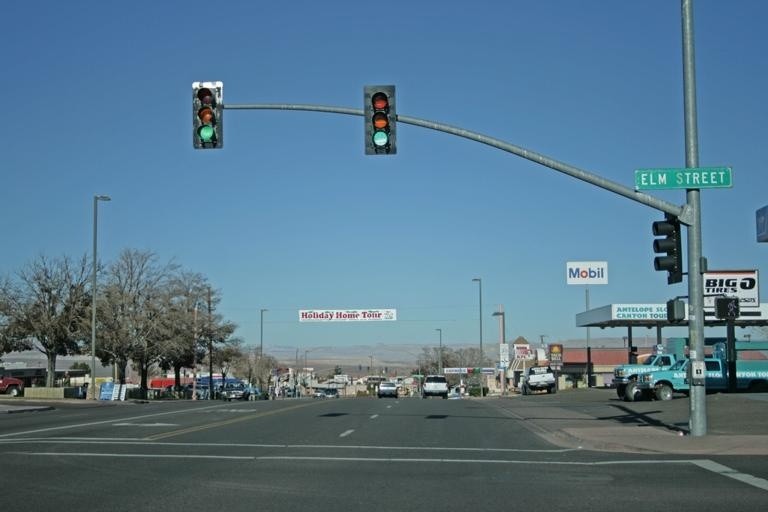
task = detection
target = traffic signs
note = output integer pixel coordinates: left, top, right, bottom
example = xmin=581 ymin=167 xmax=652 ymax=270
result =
xmin=632 ymin=165 xmax=734 ymax=192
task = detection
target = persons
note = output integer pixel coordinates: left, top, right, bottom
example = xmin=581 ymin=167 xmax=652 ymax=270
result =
xmin=64 ymin=372 xmax=70 ymax=386
xmin=31 ymin=377 xmax=40 ymax=387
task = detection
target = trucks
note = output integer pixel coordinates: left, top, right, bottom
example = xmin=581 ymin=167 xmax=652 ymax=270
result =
xmin=633 ymin=340 xmax=767 ymax=402
xmin=609 ymin=336 xmax=737 ymax=402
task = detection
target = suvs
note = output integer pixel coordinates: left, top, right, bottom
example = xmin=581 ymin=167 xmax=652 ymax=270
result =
xmin=519 ymin=362 xmax=557 ymax=396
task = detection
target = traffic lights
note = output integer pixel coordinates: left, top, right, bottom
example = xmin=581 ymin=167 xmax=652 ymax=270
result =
xmin=664 ymin=298 xmax=685 ymax=324
xmin=713 ymin=296 xmax=740 ymax=320
xmin=650 ymin=210 xmax=682 ymax=285
xmin=190 ymin=80 xmax=225 ymax=151
xmin=362 ymin=83 xmax=397 ymax=155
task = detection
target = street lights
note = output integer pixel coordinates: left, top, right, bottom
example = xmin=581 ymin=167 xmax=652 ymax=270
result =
xmin=204 ymin=284 xmax=301 ymax=401
xmin=86 ymin=193 xmax=112 ymax=403
xmin=365 ymin=274 xmax=483 ymax=401
